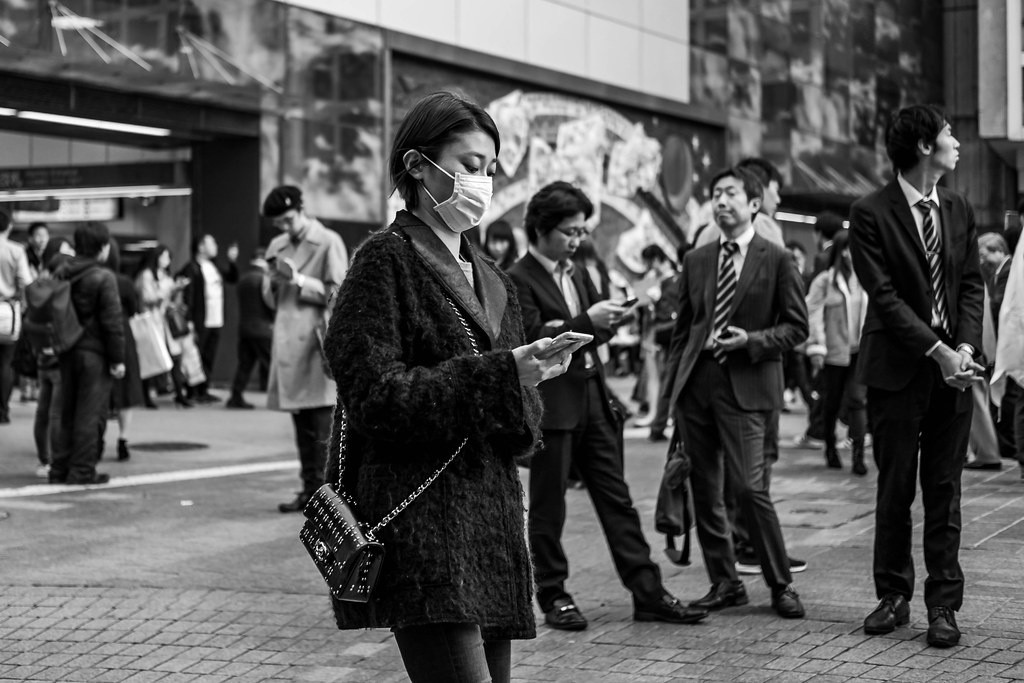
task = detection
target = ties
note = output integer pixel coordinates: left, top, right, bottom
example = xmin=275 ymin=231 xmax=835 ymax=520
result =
xmin=711 ymin=242 xmax=740 ymax=364
xmin=915 ymin=199 xmax=952 ymax=339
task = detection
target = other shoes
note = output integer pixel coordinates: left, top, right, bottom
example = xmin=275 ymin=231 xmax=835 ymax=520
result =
xmin=963 ymin=460 xmax=1003 ymax=471
xmin=141 ymin=387 xmax=254 ymax=410
xmin=36 ymin=464 xmax=50 ymax=477
xmin=49 ymin=469 xmax=110 ymax=486
xmin=118 ymin=439 xmax=131 ymax=460
xmin=825 ymin=448 xmax=842 ymax=469
xmin=733 ymin=554 xmax=808 ymax=573
xmin=850 ymin=449 xmax=867 ymax=476
xmin=279 ymin=496 xmax=307 ymax=512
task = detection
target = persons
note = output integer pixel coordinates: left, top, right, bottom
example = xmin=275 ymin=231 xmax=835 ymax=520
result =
xmin=849 ymin=103 xmax=984 ymax=645
xmin=484 ymin=181 xmax=707 ymax=630
xmin=967 ymin=217 xmax=1024 ymax=478
xmin=0 ymin=206 xmax=274 ymax=484
xmin=324 ymin=89 xmax=571 ymax=682
xmin=252 ymin=185 xmax=350 ymax=512
xmin=651 ymin=155 xmax=871 ymax=616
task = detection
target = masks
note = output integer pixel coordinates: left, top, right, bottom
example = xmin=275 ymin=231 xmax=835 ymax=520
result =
xmin=413 ymin=153 xmax=494 ymax=232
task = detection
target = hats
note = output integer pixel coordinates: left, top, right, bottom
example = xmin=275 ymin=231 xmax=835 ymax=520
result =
xmin=263 ymin=186 xmax=301 ymax=216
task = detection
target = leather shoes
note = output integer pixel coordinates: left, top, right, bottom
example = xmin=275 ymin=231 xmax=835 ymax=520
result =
xmin=545 ymin=597 xmax=587 ymax=630
xmin=772 ymin=584 xmax=805 ymax=619
xmin=863 ymin=592 xmax=909 ymax=634
xmin=632 ymin=588 xmax=709 ymax=623
xmin=928 ymin=604 xmax=960 ymax=647
xmin=689 ymin=580 xmax=750 ymax=612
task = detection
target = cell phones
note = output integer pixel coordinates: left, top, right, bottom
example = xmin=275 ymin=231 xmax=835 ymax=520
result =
xmin=266 ymin=255 xmax=277 ymax=269
xmin=533 ymin=331 xmax=595 ymax=365
xmin=718 ymin=329 xmax=732 ymax=340
xmin=615 ymin=297 xmax=639 ymax=317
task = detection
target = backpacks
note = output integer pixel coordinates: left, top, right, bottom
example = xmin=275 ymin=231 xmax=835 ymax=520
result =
xmin=26 ymin=259 xmax=98 ymax=357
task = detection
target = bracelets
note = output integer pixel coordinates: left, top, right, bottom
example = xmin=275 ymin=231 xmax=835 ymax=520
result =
xmin=960 ymin=345 xmax=973 ymax=355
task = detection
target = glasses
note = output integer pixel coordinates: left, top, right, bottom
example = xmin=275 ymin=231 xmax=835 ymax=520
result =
xmin=553 ymin=225 xmax=590 ymax=243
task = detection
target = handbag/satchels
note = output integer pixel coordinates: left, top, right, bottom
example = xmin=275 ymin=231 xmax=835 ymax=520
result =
xmin=299 ymin=487 xmax=385 ymax=602
xmin=0 ymin=297 xmax=23 ymax=343
xmin=655 ymin=420 xmax=697 ymax=564
